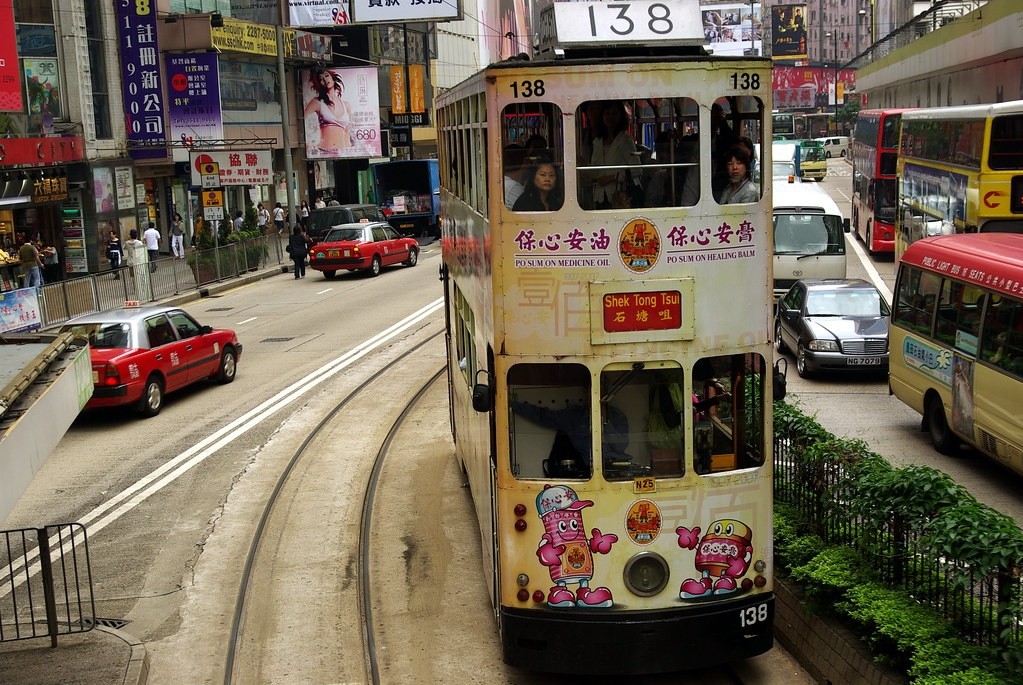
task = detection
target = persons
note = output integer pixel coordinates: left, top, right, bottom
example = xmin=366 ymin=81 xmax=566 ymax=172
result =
xmin=304 ymin=66 xmax=356 ymax=157
xmin=300 ymin=200 xmax=311 ymax=236
xmin=143 ymin=221 xmax=161 ymax=273
xmin=314 ymin=196 xmax=326 ymax=209
xmin=288 ymin=225 xmax=312 ymax=279
xmin=255 ymin=203 xmax=271 ymax=236
xmin=272 ymin=202 xmax=300 ymax=237
xmin=234 ymin=210 xmax=244 ymax=230
xmin=794 ymin=9 xmax=803 ymax=25
xmin=194 ymin=215 xmax=204 ymax=235
xmin=959 ymin=290 xmax=1022 ymax=370
xmin=644 ymin=376 xmax=734 ymax=469
xmin=122 ymin=229 xmax=143 ymax=292
xmin=327 ymin=194 xmax=340 ymax=206
xmin=0 ymin=233 xmax=60 ymax=289
xmin=901 ymin=292 xmax=957 ymax=333
xmin=105 ymin=230 xmax=124 ymax=281
xmin=366 ymin=182 xmax=375 ymax=203
xmin=314 ymin=162 xmax=322 ymax=189
xmin=224 ymin=212 xmax=234 ymax=234
xmin=514 ymin=369 xmax=629 ymax=472
xmin=168 ymin=213 xmax=187 ymax=261
xmin=774 ymin=9 xmax=788 ymax=31
xmin=504 ymin=102 xmax=764 ymax=212
xmin=105 ymin=182 xmax=114 ymax=210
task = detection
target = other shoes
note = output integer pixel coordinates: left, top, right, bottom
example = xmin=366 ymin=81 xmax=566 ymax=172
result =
xmin=151 ymin=268 xmax=157 ymax=273
xmin=114 ymin=275 xmax=120 ymax=279
xmin=277 ymin=232 xmax=281 ymax=238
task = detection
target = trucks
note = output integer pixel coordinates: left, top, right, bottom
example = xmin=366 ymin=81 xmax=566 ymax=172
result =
xmin=372 ymin=158 xmax=441 ymax=235
xmin=794 ymin=139 xmax=827 ymax=182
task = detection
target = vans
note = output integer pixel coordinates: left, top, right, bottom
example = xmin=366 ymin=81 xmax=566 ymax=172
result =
xmin=752 ymin=141 xmax=797 ymax=183
xmin=306 ymin=203 xmax=391 ymax=242
xmin=815 ymin=136 xmax=848 ymax=159
xmin=889 ymin=232 xmax=1023 ymax=480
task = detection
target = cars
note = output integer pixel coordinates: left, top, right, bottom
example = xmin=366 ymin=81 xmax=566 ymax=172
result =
xmin=309 ymin=219 xmax=420 ymax=280
xmin=55 ymin=301 xmax=243 ymax=417
xmin=776 ymin=277 xmax=892 ymax=379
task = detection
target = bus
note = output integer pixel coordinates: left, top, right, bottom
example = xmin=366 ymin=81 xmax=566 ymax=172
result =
xmin=433 ymin=37 xmax=789 ymax=678
xmin=754 ymin=160 xmax=851 ymax=314
xmin=893 ymin=101 xmax=1023 ymax=287
xmin=850 ymin=107 xmax=924 ymax=256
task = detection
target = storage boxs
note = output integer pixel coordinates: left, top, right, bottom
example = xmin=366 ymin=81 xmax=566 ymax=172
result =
xmin=391 ymin=195 xmax=408 ymax=212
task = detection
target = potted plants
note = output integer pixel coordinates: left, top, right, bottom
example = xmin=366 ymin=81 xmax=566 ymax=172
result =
xmin=185 ymin=201 xmax=269 ymax=285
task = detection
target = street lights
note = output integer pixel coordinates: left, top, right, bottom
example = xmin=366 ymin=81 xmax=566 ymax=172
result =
xmin=858 ymin=4 xmax=874 ymax=63
xmin=825 ymin=28 xmax=838 ymax=136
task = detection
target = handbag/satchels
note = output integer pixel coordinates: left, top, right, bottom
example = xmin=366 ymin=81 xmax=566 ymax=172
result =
xmin=265 ymin=221 xmax=269 ymax=229
xmin=611 ymin=168 xmax=647 ymax=209
xmin=274 ymin=220 xmax=276 ymax=225
